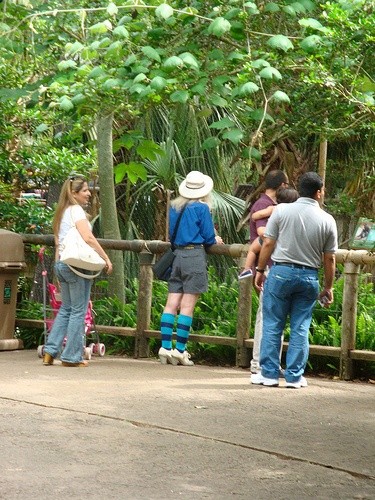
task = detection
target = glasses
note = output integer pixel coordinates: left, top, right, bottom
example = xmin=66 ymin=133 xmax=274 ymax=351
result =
xmin=279 ymin=183 xmax=290 ymax=186
xmin=70 ymin=178 xmax=74 ymax=192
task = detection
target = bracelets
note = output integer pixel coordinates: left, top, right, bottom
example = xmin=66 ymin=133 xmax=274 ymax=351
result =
xmin=255 ymin=267 xmax=264 ymax=272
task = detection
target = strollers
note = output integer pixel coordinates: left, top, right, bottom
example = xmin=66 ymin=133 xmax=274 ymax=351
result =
xmin=38 ymin=248 xmax=105 ymax=359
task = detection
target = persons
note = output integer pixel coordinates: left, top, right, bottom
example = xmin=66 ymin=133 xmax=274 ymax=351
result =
xmin=254 ymin=172 xmax=338 ymax=388
xmin=249 ymin=169 xmax=289 ymax=377
xmin=238 ymin=186 xmax=300 ymax=279
xmin=43 ymin=178 xmax=113 ymax=367
xmin=158 ymin=172 xmax=222 ymax=366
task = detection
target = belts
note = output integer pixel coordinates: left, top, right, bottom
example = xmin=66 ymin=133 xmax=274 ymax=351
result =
xmin=272 ymin=261 xmax=311 ymax=269
xmin=174 ymin=244 xmax=204 ymax=250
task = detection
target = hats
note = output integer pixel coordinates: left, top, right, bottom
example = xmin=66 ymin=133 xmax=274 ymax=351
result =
xmin=179 ymin=171 xmax=214 ymax=199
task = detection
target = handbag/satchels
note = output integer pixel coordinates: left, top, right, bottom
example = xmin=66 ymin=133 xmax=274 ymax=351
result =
xmin=60 ymin=205 xmax=106 ymax=271
xmin=153 ymin=250 xmax=176 ymax=281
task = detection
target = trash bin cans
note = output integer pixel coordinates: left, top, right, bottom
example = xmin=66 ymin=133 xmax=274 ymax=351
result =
xmin=0 ymin=228 xmax=25 ymax=350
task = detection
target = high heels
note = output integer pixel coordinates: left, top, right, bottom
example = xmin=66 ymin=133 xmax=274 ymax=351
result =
xmin=171 ymin=348 xmax=194 ymax=366
xmin=158 ymin=347 xmax=181 ymax=365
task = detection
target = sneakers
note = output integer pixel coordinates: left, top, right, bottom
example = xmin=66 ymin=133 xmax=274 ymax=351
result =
xmin=251 ymin=374 xmax=279 ymax=386
xmin=287 ymin=375 xmax=307 ymax=388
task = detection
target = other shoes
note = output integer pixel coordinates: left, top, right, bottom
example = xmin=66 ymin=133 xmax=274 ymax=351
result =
xmin=43 ymin=353 xmax=54 ymax=365
xmin=62 ymin=361 xmax=86 ymax=367
xmin=238 ymin=269 xmax=253 ymax=279
